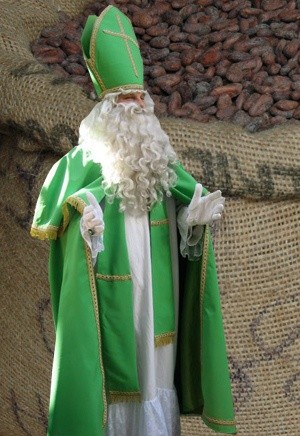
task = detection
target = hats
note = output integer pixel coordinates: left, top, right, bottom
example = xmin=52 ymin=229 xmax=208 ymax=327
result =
xmin=80 ymin=5 xmax=144 ymax=99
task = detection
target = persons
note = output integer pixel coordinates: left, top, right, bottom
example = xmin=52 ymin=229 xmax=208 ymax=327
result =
xmin=30 ymin=5 xmax=237 ymax=435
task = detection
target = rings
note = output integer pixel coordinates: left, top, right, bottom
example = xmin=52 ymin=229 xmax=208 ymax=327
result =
xmin=88 ymin=227 xmax=95 ymax=235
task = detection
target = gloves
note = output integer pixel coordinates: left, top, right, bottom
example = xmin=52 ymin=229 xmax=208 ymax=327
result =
xmin=84 ymin=191 xmax=104 ymax=237
xmin=188 ymin=183 xmax=225 ymax=225
